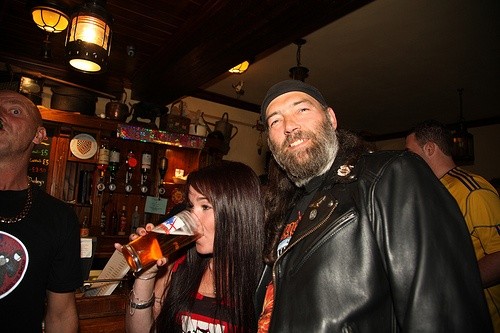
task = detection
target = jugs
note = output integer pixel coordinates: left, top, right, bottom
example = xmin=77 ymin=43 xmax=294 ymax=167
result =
xmin=202 ymin=111 xmax=239 ymax=144
xmin=105 ymin=89 xmax=135 ymax=124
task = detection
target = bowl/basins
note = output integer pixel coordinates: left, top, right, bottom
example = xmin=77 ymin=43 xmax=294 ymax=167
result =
xmin=172 ymin=176 xmax=188 ymax=184
xmin=188 ymin=123 xmax=207 ymax=137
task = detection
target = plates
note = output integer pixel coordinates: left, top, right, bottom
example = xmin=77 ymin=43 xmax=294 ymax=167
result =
xmin=69 ymin=133 xmax=98 ymax=159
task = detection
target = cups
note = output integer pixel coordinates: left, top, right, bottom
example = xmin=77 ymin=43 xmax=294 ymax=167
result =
xmin=121 ymin=207 xmax=204 ymax=272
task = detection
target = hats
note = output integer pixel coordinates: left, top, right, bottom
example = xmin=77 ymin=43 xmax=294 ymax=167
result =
xmin=261 ymin=80 xmax=327 ymax=114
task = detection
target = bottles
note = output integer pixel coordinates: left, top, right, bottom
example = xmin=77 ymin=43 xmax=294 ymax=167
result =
xmin=123 ymin=151 xmax=138 ymax=197
xmin=141 ymin=152 xmax=151 ymax=197
xmin=110 ymin=202 xmax=118 ymax=236
xmin=100 ymin=208 xmax=106 ymax=235
xmin=80 ymin=215 xmax=89 ymax=238
xmin=97 ymin=144 xmax=110 ymax=195
xmin=108 ymin=148 xmax=120 ymax=196
xmin=118 ymin=206 xmax=127 ymax=235
xmin=131 ymin=206 xmax=148 ymax=234
xmin=158 ymin=157 xmax=168 ymax=198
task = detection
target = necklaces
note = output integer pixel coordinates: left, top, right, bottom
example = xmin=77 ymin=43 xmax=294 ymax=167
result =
xmin=207 ymin=261 xmax=218 ymax=296
xmin=0 ymin=175 xmax=33 ymax=223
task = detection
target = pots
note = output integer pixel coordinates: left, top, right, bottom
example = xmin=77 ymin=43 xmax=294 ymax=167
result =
xmin=50 ymin=86 xmax=97 ymax=114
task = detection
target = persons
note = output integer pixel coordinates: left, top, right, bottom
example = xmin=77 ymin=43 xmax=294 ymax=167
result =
xmin=400 ymin=112 xmax=500 ymax=333
xmin=115 ymin=160 xmax=275 ymax=333
xmin=261 ymin=78 xmax=479 ymax=333
xmin=0 ymin=89 xmax=79 ymax=333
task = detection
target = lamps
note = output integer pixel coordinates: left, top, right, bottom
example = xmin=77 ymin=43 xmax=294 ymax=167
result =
xmin=448 ymin=89 xmax=475 ymax=166
xmin=30 ymin=0 xmax=70 ymax=34
xmin=64 ymin=0 xmax=112 ymax=74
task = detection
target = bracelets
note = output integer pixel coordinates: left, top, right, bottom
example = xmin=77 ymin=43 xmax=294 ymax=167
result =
xmin=128 ymin=289 xmax=156 ymax=315
xmin=133 ymin=272 xmax=158 ymax=281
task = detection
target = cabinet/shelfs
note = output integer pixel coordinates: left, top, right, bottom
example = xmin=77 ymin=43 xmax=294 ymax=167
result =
xmin=37 ymin=106 xmax=205 ymax=257
